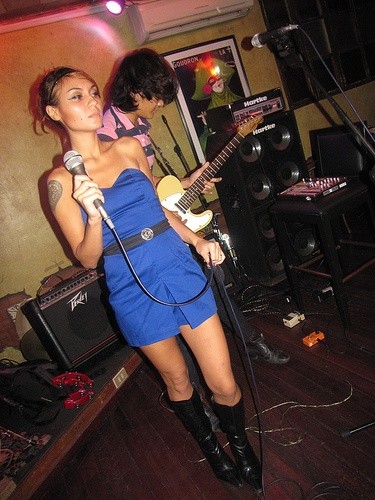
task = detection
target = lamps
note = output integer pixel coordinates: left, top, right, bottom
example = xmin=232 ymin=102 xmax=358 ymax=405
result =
xmin=106 ymin=0 xmax=125 ymax=15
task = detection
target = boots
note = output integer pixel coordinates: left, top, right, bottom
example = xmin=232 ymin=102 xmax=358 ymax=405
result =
xmin=162 ymin=384 xmax=245 ymax=489
xmin=212 ymin=393 xmax=263 ymax=491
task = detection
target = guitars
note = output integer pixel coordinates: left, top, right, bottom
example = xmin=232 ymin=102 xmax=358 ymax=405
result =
xmin=157 ymin=116 xmax=266 ymax=244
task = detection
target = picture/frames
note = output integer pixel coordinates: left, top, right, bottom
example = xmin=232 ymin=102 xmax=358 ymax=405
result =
xmin=160 ymin=34 xmax=253 ymax=166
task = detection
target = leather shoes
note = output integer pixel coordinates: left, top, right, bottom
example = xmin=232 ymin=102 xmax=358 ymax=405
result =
xmin=245 ymin=333 xmax=292 ymax=366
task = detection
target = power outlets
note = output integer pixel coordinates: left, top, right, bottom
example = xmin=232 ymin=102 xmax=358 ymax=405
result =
xmin=112 ymin=367 xmax=129 ymax=389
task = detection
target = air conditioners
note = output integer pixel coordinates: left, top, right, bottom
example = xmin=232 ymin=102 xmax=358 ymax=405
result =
xmin=126 ymin=0 xmax=254 ymax=46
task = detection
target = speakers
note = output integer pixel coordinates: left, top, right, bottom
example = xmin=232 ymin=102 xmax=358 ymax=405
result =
xmin=206 ymin=109 xmax=341 ymax=287
xmin=21 ymin=266 xmax=124 ymax=372
xmin=189 ymin=233 xmax=243 ymax=295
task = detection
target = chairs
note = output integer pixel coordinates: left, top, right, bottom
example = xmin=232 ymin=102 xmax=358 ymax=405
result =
xmin=268 ymin=120 xmax=375 ymax=329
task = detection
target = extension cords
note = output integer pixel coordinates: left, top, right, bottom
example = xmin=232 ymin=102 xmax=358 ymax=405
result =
xmin=318 ymin=287 xmax=334 ymax=303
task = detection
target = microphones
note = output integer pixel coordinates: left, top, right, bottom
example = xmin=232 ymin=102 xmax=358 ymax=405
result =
xmin=64 ymin=151 xmax=115 ymax=229
xmin=242 ymin=23 xmax=299 ymax=50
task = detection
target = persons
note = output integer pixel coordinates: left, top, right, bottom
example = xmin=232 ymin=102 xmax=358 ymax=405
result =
xmin=97 ymin=48 xmax=292 ymax=366
xmin=39 ymin=66 xmax=262 ymax=492
